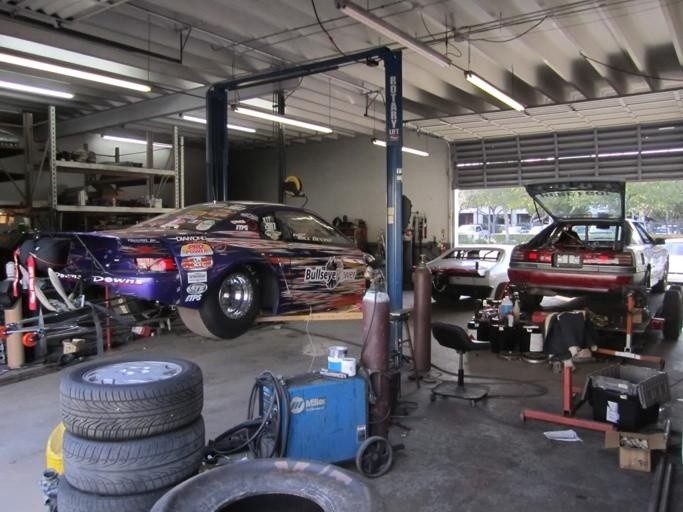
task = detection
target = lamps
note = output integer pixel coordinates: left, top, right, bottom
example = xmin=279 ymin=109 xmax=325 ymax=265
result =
xmin=336 ymin=0 xmax=451 ymax=69
xmin=0 ymin=53 xmax=151 ymax=93
xmin=464 ymin=70 xmax=527 ymax=111
xmin=371 ymin=138 xmax=429 ymax=157
xmin=179 ymin=114 xmax=256 ymax=133
xmin=231 ymin=104 xmax=332 ymax=134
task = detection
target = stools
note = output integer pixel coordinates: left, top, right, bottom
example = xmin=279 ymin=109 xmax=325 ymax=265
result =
xmin=431 ymin=323 xmax=491 ymax=408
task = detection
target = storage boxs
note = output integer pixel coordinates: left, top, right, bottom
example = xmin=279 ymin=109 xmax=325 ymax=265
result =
xmin=605 ymin=431 xmax=666 ymax=472
xmin=582 ymin=364 xmax=671 ymax=432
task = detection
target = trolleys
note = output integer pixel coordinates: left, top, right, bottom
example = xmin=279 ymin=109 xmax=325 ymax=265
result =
xmin=257 ymin=268 xmax=405 ymax=478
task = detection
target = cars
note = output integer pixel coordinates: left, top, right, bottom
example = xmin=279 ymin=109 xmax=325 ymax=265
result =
xmin=456 ymin=222 xmax=547 ymax=240
xmin=509 ymin=181 xmax=670 ymax=307
xmin=664 ymin=237 xmax=682 ymax=285
xmin=588 ymin=225 xmax=612 ymax=240
xmin=425 ymin=243 xmax=515 ymax=302
xmin=13 ymin=200 xmax=376 ymax=339
xmin=652 ymin=223 xmax=680 ymax=235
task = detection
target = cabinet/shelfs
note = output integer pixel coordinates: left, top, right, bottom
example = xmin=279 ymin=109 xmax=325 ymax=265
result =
xmin=48 ymin=106 xmax=184 ymax=214
xmin=0 ymin=113 xmax=33 ymax=208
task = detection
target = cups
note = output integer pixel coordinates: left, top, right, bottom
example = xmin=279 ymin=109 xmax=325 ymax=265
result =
xmin=340 ymin=357 xmax=356 ymax=378
xmin=76 ymin=190 xmax=86 ymax=206
xmin=154 ymin=199 xmax=162 ymax=209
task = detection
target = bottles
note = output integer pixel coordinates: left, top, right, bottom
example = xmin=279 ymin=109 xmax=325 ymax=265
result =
xmin=499 ymin=295 xmax=520 ymax=327
xmin=327 ymin=347 xmax=348 ymax=373
